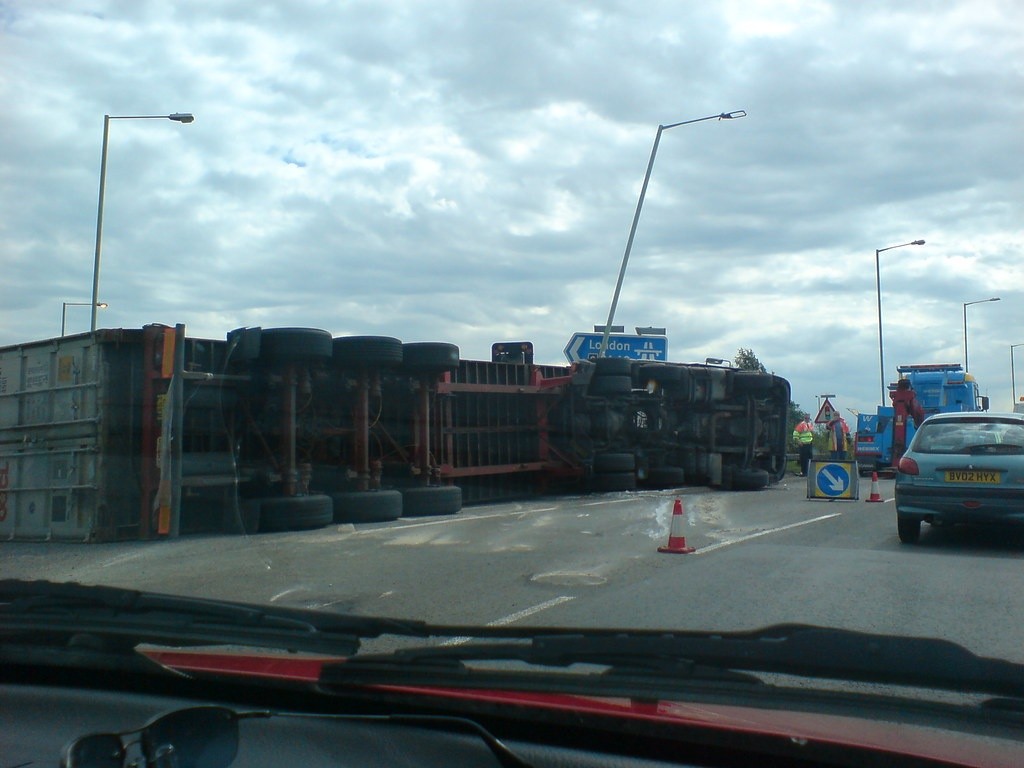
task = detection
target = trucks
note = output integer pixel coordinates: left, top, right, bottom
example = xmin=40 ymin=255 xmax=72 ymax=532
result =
xmin=852 ymin=363 xmax=989 ymax=478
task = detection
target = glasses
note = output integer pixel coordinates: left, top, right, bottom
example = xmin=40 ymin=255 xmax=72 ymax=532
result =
xmin=59 ymin=705 xmax=534 ymax=768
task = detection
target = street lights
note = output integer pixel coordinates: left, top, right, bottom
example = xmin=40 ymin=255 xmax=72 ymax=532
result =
xmin=814 ymin=395 xmax=821 ymax=435
xmin=62 ymin=302 xmax=108 ymax=335
xmin=595 ymin=108 xmax=751 ymax=358
xmin=875 ymin=240 xmax=926 ymax=405
xmin=963 ymin=297 xmax=1001 ymax=374
xmin=90 ymin=111 xmax=195 ymax=328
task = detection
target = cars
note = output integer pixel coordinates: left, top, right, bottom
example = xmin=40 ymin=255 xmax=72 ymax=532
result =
xmin=893 ymin=410 xmax=1024 ymax=544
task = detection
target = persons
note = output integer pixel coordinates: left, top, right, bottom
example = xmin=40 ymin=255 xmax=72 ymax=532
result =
xmin=792 ymin=413 xmax=814 ymax=476
xmin=826 ymin=412 xmax=852 ymax=461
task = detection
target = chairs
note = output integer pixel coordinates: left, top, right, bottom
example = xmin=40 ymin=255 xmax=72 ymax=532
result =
xmin=941 ymin=426 xmax=964 ymax=446
xmin=1002 ymin=429 xmax=1024 ymax=446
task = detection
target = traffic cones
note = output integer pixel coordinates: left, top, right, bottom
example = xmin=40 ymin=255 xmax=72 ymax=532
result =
xmin=865 ymin=472 xmax=885 ymax=504
xmin=658 ymin=497 xmax=696 ymax=553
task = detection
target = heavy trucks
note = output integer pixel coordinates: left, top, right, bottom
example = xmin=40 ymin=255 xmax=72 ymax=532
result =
xmin=0 ymin=324 xmax=793 ymax=542
